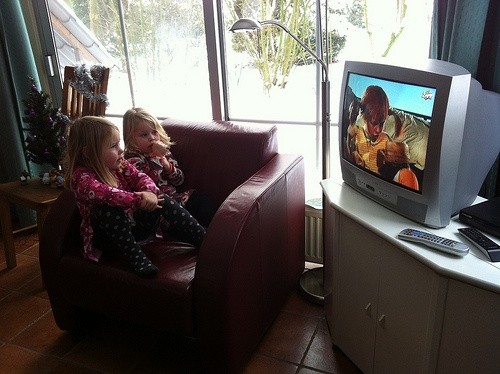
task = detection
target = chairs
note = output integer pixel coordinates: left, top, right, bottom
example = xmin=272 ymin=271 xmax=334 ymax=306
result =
xmin=0 ymin=65 xmax=110 ymax=271
xmin=37 ymin=117 xmax=305 ymax=374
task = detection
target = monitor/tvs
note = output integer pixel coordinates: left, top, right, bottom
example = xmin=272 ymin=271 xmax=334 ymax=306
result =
xmin=337 ymin=58 xmax=500 ymax=228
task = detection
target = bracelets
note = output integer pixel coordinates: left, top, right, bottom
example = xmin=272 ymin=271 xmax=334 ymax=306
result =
xmin=167 ymin=163 xmax=173 ymax=173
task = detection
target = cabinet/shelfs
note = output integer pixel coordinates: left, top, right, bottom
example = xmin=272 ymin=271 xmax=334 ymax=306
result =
xmin=320 ymin=178 xmax=500 ymax=374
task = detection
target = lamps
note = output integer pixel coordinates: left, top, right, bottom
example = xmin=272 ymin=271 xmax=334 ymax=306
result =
xmin=228 ymin=18 xmax=331 ymax=308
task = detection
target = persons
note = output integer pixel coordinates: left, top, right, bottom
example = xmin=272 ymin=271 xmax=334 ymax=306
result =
xmin=347 ymin=85 xmax=420 ymax=191
xmin=122 ymin=108 xmax=216 ymax=228
xmin=63 ymin=116 xmax=207 ymax=279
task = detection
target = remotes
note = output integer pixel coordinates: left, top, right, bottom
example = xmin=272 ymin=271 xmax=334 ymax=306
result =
xmin=458 ymin=228 xmax=500 ymax=261
xmin=398 ymin=229 xmax=469 ymax=256
